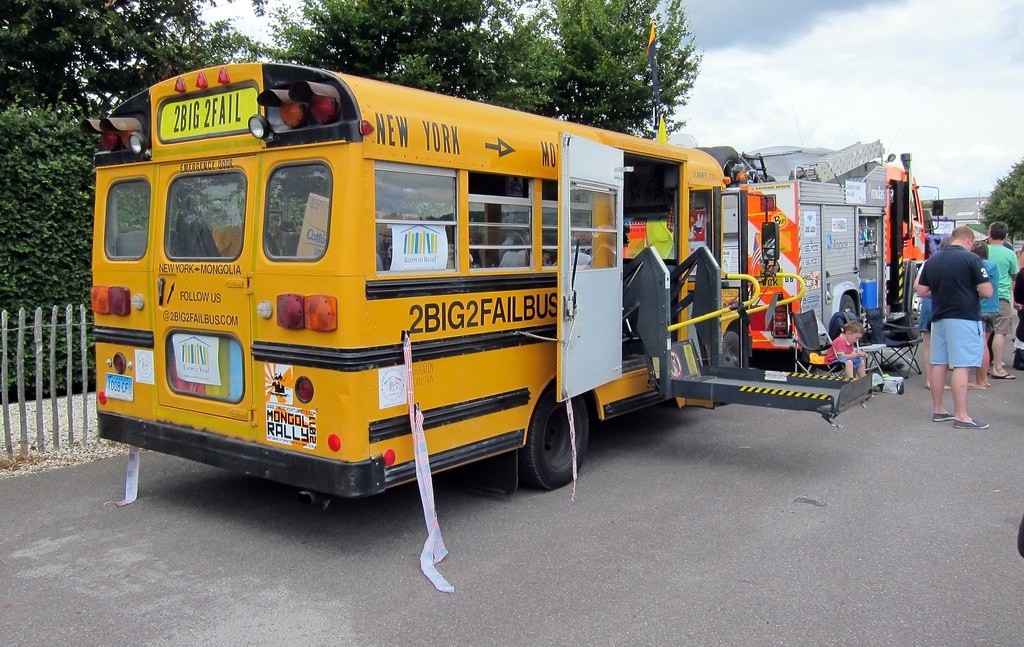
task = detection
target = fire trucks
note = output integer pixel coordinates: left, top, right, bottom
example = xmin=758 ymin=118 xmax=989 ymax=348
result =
xmin=722 ymin=140 xmax=944 ymax=367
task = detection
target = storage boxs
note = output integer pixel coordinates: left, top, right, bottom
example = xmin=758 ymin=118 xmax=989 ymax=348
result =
xmin=860 ymin=278 xmax=877 ymax=310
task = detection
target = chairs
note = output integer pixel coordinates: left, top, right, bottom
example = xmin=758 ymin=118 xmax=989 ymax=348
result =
xmin=789 ymin=306 xmax=923 ymax=381
xmin=114 ymin=225 xmax=300 ymax=257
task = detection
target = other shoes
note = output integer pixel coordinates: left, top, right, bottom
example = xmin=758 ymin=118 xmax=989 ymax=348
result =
xmin=925 ymin=381 xmax=951 ymax=390
xmin=967 ymin=381 xmax=986 ymax=390
xmin=954 ymin=417 xmax=990 ymax=428
xmin=932 ymin=411 xmax=955 ymax=421
xmin=991 ymin=359 xmax=1005 ymax=367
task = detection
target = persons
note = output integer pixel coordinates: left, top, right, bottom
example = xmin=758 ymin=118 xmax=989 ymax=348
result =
xmin=913 ymin=220 xmax=1024 ymax=391
xmin=824 ymin=322 xmax=868 ymax=378
xmin=916 ymin=226 xmax=994 ymax=430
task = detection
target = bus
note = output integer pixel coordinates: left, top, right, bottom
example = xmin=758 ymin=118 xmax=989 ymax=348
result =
xmin=81 ymin=62 xmax=779 ymax=510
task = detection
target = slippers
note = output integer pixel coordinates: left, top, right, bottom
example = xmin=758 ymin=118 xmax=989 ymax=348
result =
xmin=991 ymin=372 xmax=1016 ymax=380
